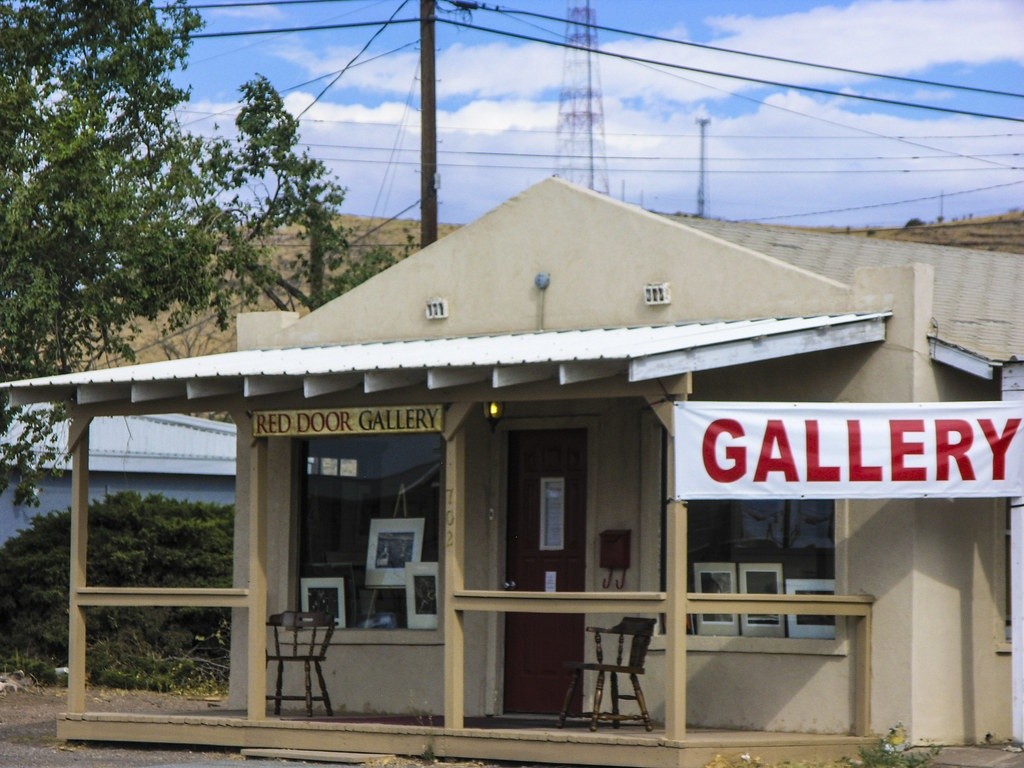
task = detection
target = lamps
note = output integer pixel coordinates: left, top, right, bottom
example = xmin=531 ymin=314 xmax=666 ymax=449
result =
xmin=482 ymin=399 xmax=504 ymax=433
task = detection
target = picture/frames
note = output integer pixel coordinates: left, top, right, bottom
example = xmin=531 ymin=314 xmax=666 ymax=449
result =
xmin=783 ymin=578 xmax=836 ymax=638
xmin=693 ymin=561 xmax=740 ymax=636
xmin=403 ymin=560 xmax=440 ymax=630
xmin=298 ymin=575 xmax=347 ymax=631
xmin=739 ymin=562 xmax=786 ymax=636
xmin=364 ymin=519 xmax=426 ymax=587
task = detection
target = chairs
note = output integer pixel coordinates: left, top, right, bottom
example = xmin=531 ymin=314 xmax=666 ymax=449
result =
xmin=264 ymin=611 xmax=339 ymax=715
xmin=560 ymin=616 xmax=658 ymax=733
